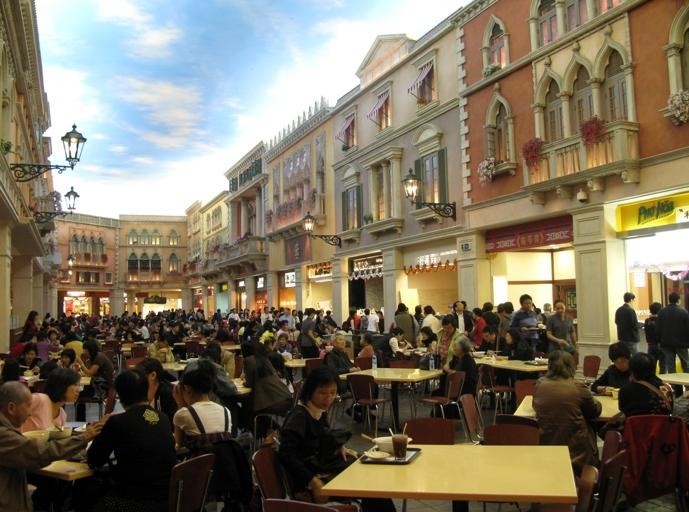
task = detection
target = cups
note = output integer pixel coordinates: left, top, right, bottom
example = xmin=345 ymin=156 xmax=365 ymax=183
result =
xmin=392 ymin=434 xmax=408 ymax=460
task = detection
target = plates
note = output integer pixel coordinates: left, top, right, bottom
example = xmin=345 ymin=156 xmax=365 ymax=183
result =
xmin=73 ymin=427 xmax=86 ymax=434
xmin=359 ymin=446 xmax=422 ymax=464
xmin=364 ymin=451 xmax=391 ymax=460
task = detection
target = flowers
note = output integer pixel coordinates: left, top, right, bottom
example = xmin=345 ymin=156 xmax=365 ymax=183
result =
xmin=579 ymin=115 xmax=607 ymax=149
xmin=668 ymin=89 xmax=689 ymax=125
xmin=478 ymin=158 xmax=495 ymax=187
xmin=275 ymin=197 xmax=301 ymax=218
xmin=522 ymin=137 xmax=544 ymax=174
xmin=309 ymin=188 xmax=317 ymax=202
xmin=263 ymin=210 xmax=272 ymax=226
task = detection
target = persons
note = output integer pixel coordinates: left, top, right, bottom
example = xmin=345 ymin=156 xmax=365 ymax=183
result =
xmin=40 ymin=308 xmax=339 ymax=342
xmin=356 ymin=331 xmax=375 ymax=358
xmin=12 ymin=342 xmax=44 ymax=376
xmin=239 ymin=340 xmax=293 ymax=437
xmin=430 ymin=336 xmax=478 ymax=418
xmin=44 ymin=329 xmax=59 ymax=344
xmin=434 ymin=315 xmax=465 ymax=391
xmin=378 ymin=327 xmax=411 ymax=360
xmin=144 ymin=336 xmax=177 ymax=364
xmin=87 ymin=369 xmax=177 ymax=511
xmin=347 ymin=303 xmax=512 ymax=333
xmin=169 ymin=359 xmax=232 ymax=498
xmin=274 ymin=334 xmax=294 ymax=359
xmin=276 ymin=364 xmax=396 ymax=511
xmin=510 ymin=295 xmax=543 ymax=361
xmin=34 ymin=334 xmax=62 ymax=360
xmin=75 ymin=341 xmax=114 ymax=422
xmin=618 ymin=352 xmax=675 ymax=416
xmin=615 ymin=292 xmax=646 ymax=354
xmin=60 ymin=332 xmax=84 ymax=358
xmin=493 ymin=328 xmax=534 ymax=361
xmin=324 ymin=334 xmax=379 ymax=425
xmin=9 ymin=337 xmax=30 ymax=357
xmin=419 ymin=327 xmax=438 ymax=347
xmin=17 ymin=368 xmax=84 ymax=433
xmin=135 ymin=359 xmax=182 ymax=418
xmin=655 ymin=291 xmax=689 ymax=393
xmin=547 ymin=299 xmax=576 ymax=352
xmin=204 ymin=344 xmax=236 ymax=382
xmin=46 ymin=349 xmax=85 ymax=378
xmin=543 ymin=302 xmax=553 ymax=317
xmin=24 ymin=311 xmax=41 ymax=340
xmin=472 ymin=328 xmax=508 ymax=357
xmin=591 ymin=342 xmax=673 ymax=398
xmin=532 ymin=350 xmax=602 ymax=474
xmin=644 ymin=302 xmax=665 ymax=374
xmin=0 ymin=379 xmax=104 ymax=511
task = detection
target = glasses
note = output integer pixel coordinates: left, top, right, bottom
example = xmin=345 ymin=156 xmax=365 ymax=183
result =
xmin=316 ymin=390 xmax=337 ymax=398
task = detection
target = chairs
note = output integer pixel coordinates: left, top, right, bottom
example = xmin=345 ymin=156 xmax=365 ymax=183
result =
xmin=625 ymin=415 xmax=689 ymax=505
xmin=515 ymin=381 xmax=538 ymax=410
xmin=306 ymin=359 xmax=353 ymax=430
xmin=252 ymin=447 xmax=361 ymax=512
xmin=478 ymin=425 xmax=540 ymax=512
xmin=355 ymin=356 xmax=372 ymax=370
xmin=496 ymin=414 xmax=539 ymax=426
xmin=252 ymin=379 xmax=304 ymax=447
xmin=417 ymin=372 xmax=468 ymax=443
xmin=578 ymin=429 xmax=627 ymax=512
xmin=576 ymin=356 xmax=601 ymax=386
xmin=186 ymin=341 xmax=199 ymax=359
xmin=75 ymin=342 xmax=122 ymax=423
xmin=474 ymin=365 xmax=514 ymax=424
xmin=402 ymin=419 xmax=455 ymax=512
xmin=347 ymin=374 xmax=397 ymax=438
xmin=457 ymin=394 xmax=485 ymax=443
xmin=382 ymin=359 xmax=418 ymax=421
xmin=172 ymin=454 xmax=218 ymax=512
xmin=235 ymin=356 xmax=243 ymax=377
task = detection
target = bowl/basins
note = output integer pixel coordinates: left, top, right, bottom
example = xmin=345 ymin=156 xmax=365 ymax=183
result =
xmin=536 ymin=358 xmax=548 ymax=364
xmin=48 ymin=426 xmax=72 ymax=440
xmin=371 ymin=436 xmax=413 ymax=453
xmin=472 ymin=351 xmax=485 ymax=358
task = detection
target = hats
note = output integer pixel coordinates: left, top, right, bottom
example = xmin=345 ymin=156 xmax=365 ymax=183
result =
xmin=503 ymin=303 xmax=513 ymax=311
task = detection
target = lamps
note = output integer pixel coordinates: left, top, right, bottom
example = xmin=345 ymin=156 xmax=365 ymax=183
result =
xmin=67 ymin=254 xmax=74 ymax=267
xmin=403 ymin=168 xmax=456 ymax=222
xmin=303 ymin=212 xmax=341 ymax=249
xmin=11 ymin=125 xmax=87 ymax=186
xmin=33 ymin=185 xmax=78 ymax=224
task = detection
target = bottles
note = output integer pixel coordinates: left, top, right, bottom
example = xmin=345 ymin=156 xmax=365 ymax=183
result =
xmin=430 ymin=355 xmax=434 ymax=371
xmin=372 ymin=355 xmax=377 ymax=371
